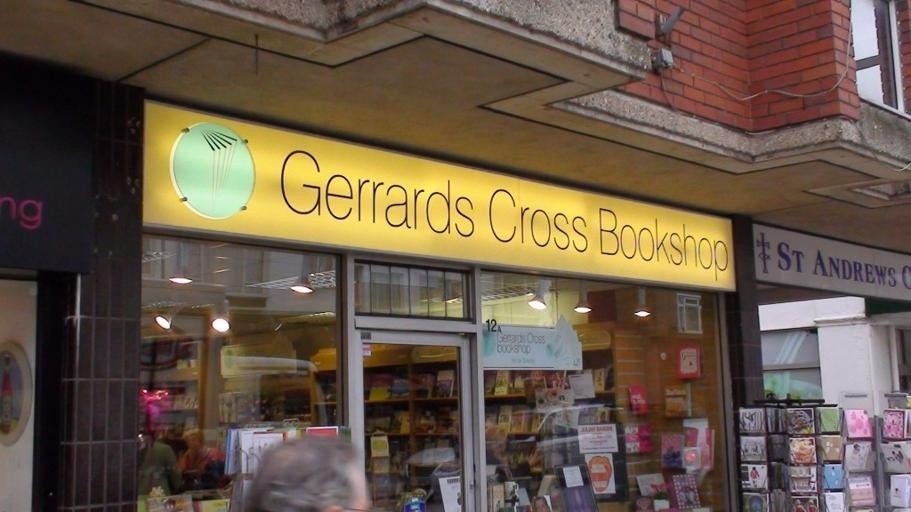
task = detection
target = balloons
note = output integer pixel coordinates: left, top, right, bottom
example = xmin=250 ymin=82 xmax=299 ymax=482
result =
xmin=139 ymin=387 xmax=171 ymax=439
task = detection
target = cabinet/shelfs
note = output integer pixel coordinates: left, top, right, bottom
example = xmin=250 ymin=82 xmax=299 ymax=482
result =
xmin=136 ymin=319 xmax=310 ymax=473
xmin=310 ymin=325 xmax=625 ymax=512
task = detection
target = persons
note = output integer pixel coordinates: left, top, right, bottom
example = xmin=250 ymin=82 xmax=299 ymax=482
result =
xmin=252 ymin=437 xmax=370 ymax=512
xmin=136 ymin=427 xmax=184 ymax=499
xmin=175 ymin=427 xmax=230 ymax=490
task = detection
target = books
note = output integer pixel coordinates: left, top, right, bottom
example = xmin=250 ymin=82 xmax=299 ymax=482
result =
xmin=738 ymin=392 xmax=911 ymax=512
xmin=221 ymin=423 xmax=340 ymax=511
xmin=363 ymin=369 xmax=717 ymax=511
xmin=137 ymin=489 xmax=233 ymax=512
xmin=675 ymin=343 xmax=700 ymax=379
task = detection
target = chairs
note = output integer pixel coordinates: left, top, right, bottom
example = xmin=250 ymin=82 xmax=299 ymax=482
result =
xmin=736 ymin=390 xmax=909 ymax=512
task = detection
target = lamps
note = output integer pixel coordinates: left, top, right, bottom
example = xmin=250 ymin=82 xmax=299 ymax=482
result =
xmin=289 ymin=248 xmax=315 ymax=294
xmin=526 ymin=279 xmax=552 ymax=310
xmin=152 ymin=299 xmax=187 ymax=330
xmin=573 ymin=279 xmax=593 ymax=314
xmin=632 ymin=285 xmax=653 ymax=317
xmin=206 ymin=284 xmax=233 ymax=333
